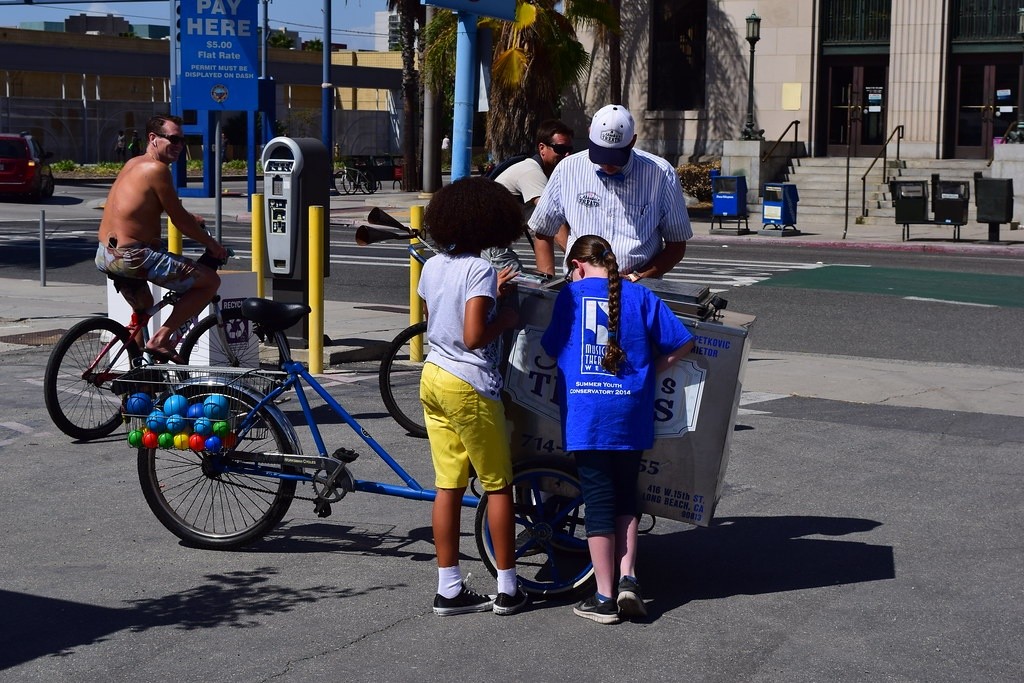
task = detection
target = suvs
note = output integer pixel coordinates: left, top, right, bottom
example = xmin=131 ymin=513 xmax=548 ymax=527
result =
xmin=0 ymin=131 xmax=55 ymax=204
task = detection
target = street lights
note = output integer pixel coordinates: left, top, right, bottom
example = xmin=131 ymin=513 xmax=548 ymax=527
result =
xmin=737 ymin=10 xmax=766 ymax=141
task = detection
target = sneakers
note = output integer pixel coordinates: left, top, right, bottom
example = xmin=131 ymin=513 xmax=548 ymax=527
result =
xmin=433 ymin=581 xmax=499 ymax=616
xmin=492 ymin=587 xmax=529 ymax=614
xmin=573 ymin=597 xmax=620 ymax=624
xmin=616 ymin=579 xmax=648 ymax=617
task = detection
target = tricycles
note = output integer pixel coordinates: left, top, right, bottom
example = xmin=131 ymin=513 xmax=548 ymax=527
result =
xmin=113 ymin=205 xmax=759 ymax=600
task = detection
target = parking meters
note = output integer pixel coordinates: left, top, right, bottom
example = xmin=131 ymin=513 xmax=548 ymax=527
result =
xmin=261 ymin=138 xmax=334 ymax=348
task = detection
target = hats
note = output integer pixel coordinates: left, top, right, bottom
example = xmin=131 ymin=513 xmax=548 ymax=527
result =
xmin=589 ymin=104 xmax=634 ymax=165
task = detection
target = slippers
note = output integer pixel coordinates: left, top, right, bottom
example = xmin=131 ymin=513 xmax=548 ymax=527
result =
xmin=139 ymin=344 xmax=186 ymax=364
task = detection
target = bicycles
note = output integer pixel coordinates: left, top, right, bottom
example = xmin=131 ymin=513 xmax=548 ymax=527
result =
xmin=335 ymin=164 xmax=379 ymax=194
xmin=43 ymin=226 xmax=290 ymax=441
xmin=377 ymin=323 xmax=431 ymax=443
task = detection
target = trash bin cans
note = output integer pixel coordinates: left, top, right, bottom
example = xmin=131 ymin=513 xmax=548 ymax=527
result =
xmin=161 ymin=270 xmax=259 ymax=370
xmin=107 ymin=272 xmax=160 ymax=371
xmin=343 ymin=154 xmax=402 ymax=181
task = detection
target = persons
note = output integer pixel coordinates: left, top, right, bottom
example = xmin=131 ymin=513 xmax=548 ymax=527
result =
xmin=440 ymin=135 xmax=451 ymax=151
xmin=222 ymin=133 xmax=228 ymax=163
xmin=114 ymin=129 xmax=142 ymax=162
xmin=541 ymin=234 xmax=696 ymax=625
xmin=527 ymin=104 xmax=693 ymax=279
xmin=480 ymin=119 xmax=574 ymax=274
xmin=417 ymin=176 xmax=533 ymax=616
xmin=93 ymin=113 xmax=228 ymax=366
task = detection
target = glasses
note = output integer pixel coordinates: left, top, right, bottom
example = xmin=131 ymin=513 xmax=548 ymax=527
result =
xmin=153 ymin=132 xmax=186 ymax=144
xmin=563 ymin=266 xmax=576 ymax=283
xmin=546 ymin=144 xmax=574 ymax=154
